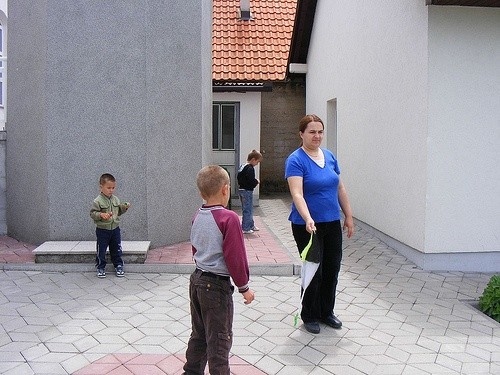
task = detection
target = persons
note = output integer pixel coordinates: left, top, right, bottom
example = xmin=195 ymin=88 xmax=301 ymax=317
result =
xmin=90 ymin=173 xmax=131 ymax=278
xmin=285 ymin=114 xmax=353 ymax=334
xmin=181 ymin=165 xmax=254 ymax=375
xmin=237 ymin=150 xmax=263 ymax=233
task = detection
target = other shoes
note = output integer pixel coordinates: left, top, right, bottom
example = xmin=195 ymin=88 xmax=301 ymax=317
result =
xmin=242 ymin=229 xmax=254 ymax=233
xmin=115 ymin=265 xmax=125 ymax=276
xmin=97 ymin=268 xmax=106 ymax=278
xmin=320 ymin=313 xmax=342 ymax=327
xmin=304 ymin=321 xmax=320 ymax=333
xmin=252 ymin=225 xmax=260 ymax=230
